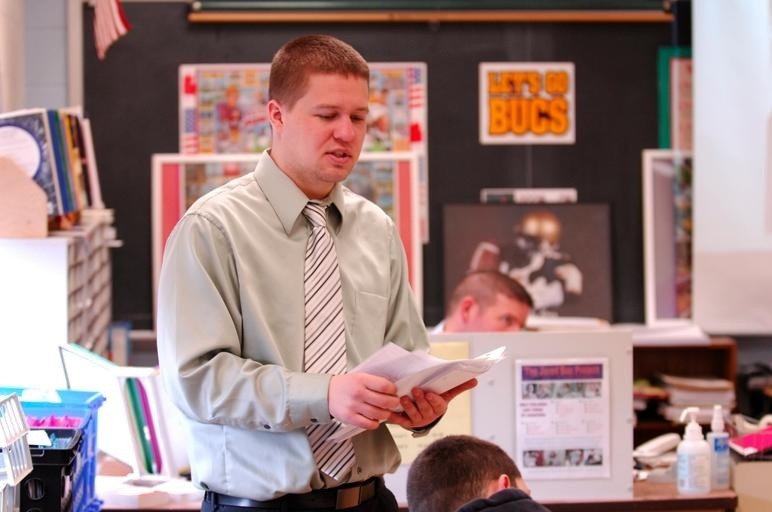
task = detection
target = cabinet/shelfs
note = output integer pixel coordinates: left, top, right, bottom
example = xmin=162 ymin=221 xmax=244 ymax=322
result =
xmin=0 ymin=222 xmax=114 ymax=366
xmin=629 ymin=335 xmax=738 ymax=449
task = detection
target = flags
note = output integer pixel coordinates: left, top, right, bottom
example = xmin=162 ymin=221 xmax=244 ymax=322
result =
xmin=89 ymin=1 xmax=133 ymax=63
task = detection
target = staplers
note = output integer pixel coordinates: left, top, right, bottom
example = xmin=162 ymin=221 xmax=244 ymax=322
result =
xmin=633 ymin=433 xmax=681 ymax=469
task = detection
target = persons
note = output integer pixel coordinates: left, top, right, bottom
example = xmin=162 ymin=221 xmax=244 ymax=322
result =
xmin=157 ymin=34 xmax=476 ymax=511
xmin=430 ymin=268 xmax=535 ymax=332
xmin=408 ymin=434 xmax=550 ymax=511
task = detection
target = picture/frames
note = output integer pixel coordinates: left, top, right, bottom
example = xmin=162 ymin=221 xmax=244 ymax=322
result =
xmin=437 ymin=199 xmax=618 ymax=325
xmin=641 ymin=40 xmax=691 ymax=332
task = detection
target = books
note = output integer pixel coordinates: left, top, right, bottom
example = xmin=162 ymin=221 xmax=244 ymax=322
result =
xmin=0 ymin=102 xmax=102 ymax=224
xmin=631 ymin=371 xmax=737 ymax=429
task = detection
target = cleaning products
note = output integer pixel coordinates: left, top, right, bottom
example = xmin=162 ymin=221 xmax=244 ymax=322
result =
xmin=679 ymin=408 xmax=708 ymax=494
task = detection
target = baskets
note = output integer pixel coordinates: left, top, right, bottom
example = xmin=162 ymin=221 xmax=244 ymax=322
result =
xmin=0 ymin=387 xmax=106 ymax=512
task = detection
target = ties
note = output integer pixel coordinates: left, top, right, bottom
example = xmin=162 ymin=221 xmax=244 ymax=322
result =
xmin=301 ymin=200 xmax=356 ymax=480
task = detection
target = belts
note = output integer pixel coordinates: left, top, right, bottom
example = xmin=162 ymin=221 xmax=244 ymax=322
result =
xmin=203 ymin=480 xmax=379 ymax=511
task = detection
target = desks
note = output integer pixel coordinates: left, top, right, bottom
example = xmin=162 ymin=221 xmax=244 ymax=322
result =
xmin=533 ymin=448 xmax=740 ymax=510
